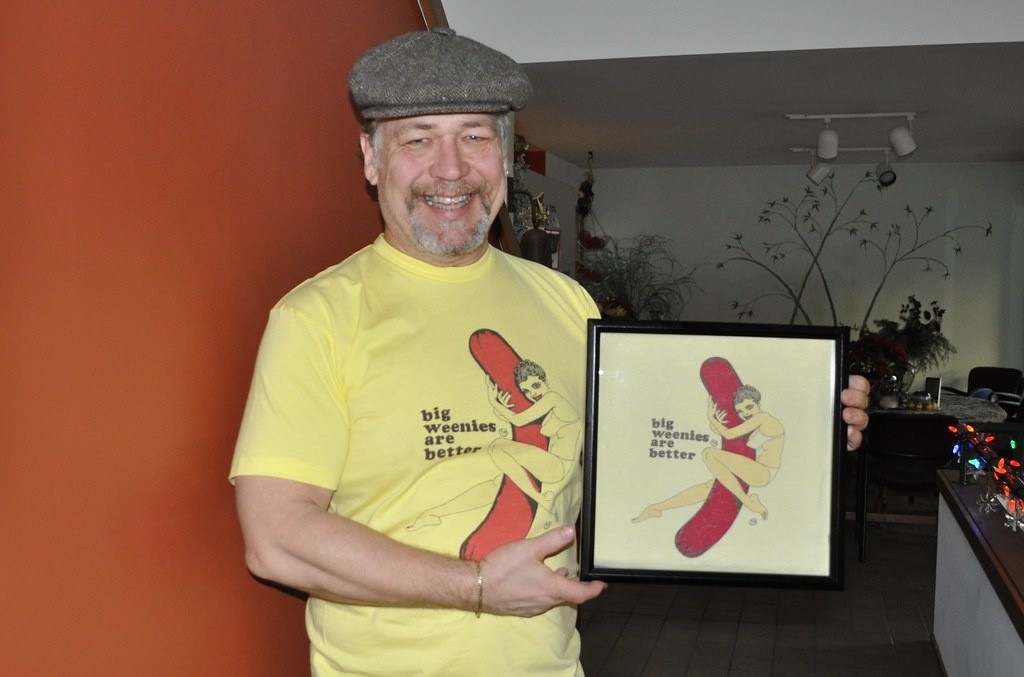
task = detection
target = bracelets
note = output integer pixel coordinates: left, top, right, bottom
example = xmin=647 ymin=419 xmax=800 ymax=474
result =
xmin=473 ymin=560 xmax=483 ymax=618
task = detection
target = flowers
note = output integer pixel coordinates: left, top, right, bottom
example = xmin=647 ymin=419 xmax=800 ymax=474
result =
xmin=849 ymin=334 xmax=916 ymax=395
xmin=873 ymin=294 xmax=957 ymax=372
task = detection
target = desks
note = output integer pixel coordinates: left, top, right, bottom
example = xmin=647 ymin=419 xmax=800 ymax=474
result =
xmin=930 ymin=469 xmax=1024 ymax=677
xmin=867 ymin=394 xmax=1008 ymax=506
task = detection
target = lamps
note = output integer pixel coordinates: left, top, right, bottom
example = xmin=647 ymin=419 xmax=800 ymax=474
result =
xmin=806 ymin=155 xmax=832 ymax=186
xmin=817 ymin=124 xmax=839 ymax=160
xmin=888 ymin=121 xmax=918 ymax=159
xmin=876 ymin=163 xmax=897 ymax=186
xmin=507 ymin=176 xmax=552 ymax=268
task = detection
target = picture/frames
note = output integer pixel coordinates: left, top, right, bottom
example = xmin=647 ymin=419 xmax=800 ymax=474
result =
xmin=577 ymin=317 xmax=844 ymax=592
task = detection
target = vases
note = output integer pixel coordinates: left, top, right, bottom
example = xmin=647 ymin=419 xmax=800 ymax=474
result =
xmin=887 ymin=365 xmax=915 ymax=392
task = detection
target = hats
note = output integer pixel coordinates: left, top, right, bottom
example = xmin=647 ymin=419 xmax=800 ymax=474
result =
xmin=347 ymin=26 xmax=534 ymax=119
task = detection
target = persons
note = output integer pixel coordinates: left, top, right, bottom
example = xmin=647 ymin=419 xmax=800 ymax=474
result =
xmin=228 ymin=25 xmax=870 ymax=677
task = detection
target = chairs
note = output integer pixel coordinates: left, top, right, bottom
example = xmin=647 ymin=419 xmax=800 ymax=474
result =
xmin=941 ymin=367 xmax=1024 ymax=418
xmin=946 ymin=397 xmax=1024 ymax=471
